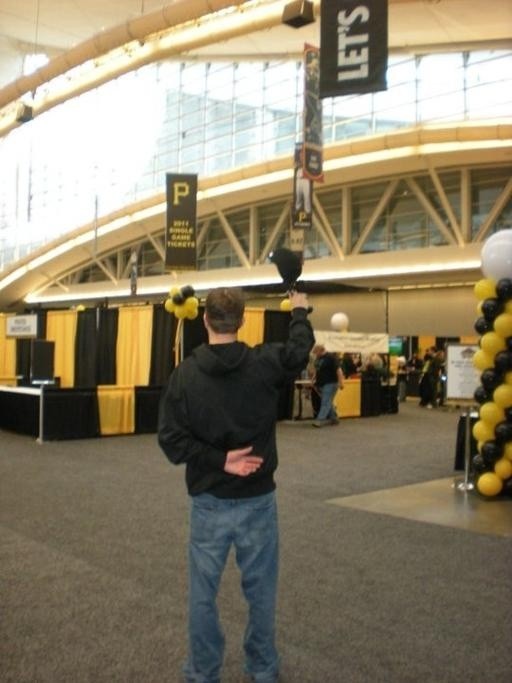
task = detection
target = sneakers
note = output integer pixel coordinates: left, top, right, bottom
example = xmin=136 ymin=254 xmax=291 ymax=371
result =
xmin=312 ymin=421 xmax=320 ymax=428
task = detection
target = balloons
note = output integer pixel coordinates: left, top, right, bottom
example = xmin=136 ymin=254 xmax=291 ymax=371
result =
xmin=472 ymin=229 xmax=512 ymax=497
xmin=330 ymin=312 xmax=348 ymax=330
xmin=164 ymin=285 xmax=199 ymax=320
xmin=280 ymin=298 xmax=292 ymax=311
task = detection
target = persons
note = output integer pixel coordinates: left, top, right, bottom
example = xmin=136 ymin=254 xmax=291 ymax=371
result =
xmin=158 ymin=286 xmax=316 ymax=683
xmin=397 ymin=345 xmax=445 ymax=408
xmin=311 ymin=344 xmax=345 ymax=428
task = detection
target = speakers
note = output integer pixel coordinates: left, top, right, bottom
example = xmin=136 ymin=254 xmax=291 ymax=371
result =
xmin=16 ymin=104 xmax=32 ymax=123
xmin=282 ymin=0 xmax=314 ymax=29
